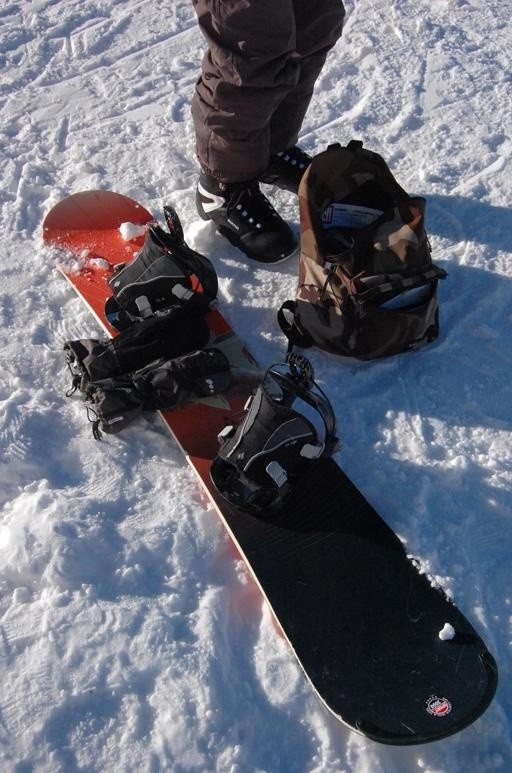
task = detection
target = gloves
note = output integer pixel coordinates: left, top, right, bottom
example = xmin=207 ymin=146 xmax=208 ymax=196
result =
xmin=64 ymin=304 xmax=232 ymax=441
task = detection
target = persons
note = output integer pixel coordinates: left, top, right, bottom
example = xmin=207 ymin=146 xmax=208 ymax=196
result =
xmin=190 ymin=0 xmax=346 ymax=264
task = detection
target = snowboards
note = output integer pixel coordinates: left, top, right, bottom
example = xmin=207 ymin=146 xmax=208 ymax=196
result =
xmin=42 ymin=190 xmax=498 ymax=746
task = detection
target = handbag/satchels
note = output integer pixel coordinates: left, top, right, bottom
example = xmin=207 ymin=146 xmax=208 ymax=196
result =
xmin=291 ymin=140 xmax=447 ymax=361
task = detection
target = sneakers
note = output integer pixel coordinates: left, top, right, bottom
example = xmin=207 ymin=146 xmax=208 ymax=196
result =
xmin=195 ymin=171 xmax=298 ymax=266
xmin=259 ymin=143 xmax=313 ymax=195
